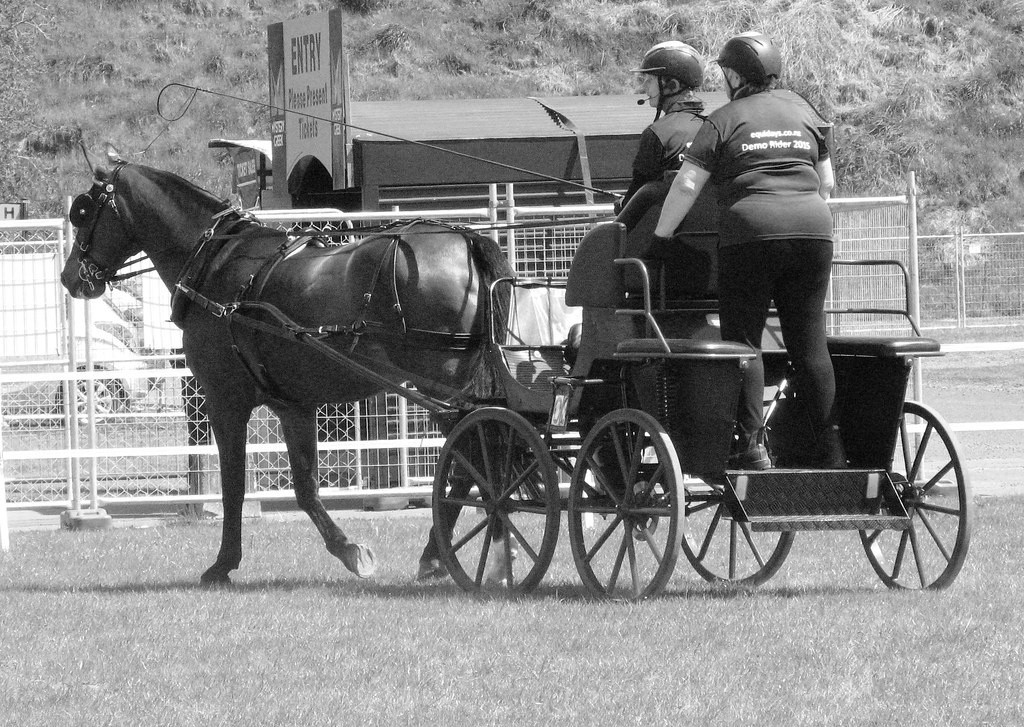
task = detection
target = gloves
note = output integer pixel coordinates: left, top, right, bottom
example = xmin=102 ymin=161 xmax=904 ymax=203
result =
xmin=614 ymin=195 xmax=625 ymax=215
xmin=650 ymin=232 xmax=677 ymax=258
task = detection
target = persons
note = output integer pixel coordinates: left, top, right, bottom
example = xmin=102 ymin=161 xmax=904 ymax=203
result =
xmin=639 ymin=30 xmax=846 ymax=470
xmin=567 ymin=39 xmax=709 ymax=365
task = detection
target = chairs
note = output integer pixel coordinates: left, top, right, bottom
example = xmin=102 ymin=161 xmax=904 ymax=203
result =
xmin=566 ymin=171 xmax=725 ymax=308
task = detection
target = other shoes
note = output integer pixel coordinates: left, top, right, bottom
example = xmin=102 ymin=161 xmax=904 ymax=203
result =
xmin=728 ymin=420 xmax=773 ymax=470
xmin=567 ymin=323 xmax=582 ymax=360
xmin=795 ymin=423 xmax=850 ymax=469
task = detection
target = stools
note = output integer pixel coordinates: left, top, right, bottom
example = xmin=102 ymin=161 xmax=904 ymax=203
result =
xmin=826 ymin=336 xmax=945 ymax=470
xmin=615 ymin=336 xmax=757 ymax=473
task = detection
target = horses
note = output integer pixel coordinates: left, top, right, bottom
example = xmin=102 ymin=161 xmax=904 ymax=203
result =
xmin=59 ymin=143 xmax=513 ymax=586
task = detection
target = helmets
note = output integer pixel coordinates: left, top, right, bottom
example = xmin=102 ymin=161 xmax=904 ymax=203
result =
xmin=709 ymin=31 xmax=781 ymax=82
xmin=630 ymin=41 xmax=704 ymax=87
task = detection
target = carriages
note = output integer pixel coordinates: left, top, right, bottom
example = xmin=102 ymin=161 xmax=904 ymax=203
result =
xmin=60 ymin=141 xmax=973 ymax=605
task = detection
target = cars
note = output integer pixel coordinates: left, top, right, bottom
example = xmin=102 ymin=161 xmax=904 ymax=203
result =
xmin=0 ymin=283 xmax=148 ymax=426
